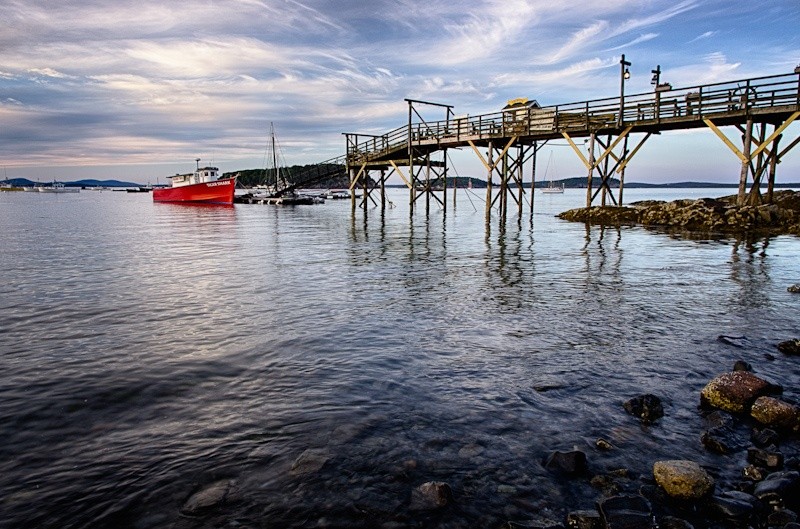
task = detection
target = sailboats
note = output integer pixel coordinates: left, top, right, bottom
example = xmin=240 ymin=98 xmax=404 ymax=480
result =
xmin=249 ymin=121 xmax=298 ymax=197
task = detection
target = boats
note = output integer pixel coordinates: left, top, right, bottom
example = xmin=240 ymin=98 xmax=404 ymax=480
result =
xmin=153 ymin=156 xmax=237 ymax=205
xmin=0 ymin=178 xmax=163 ymax=194
